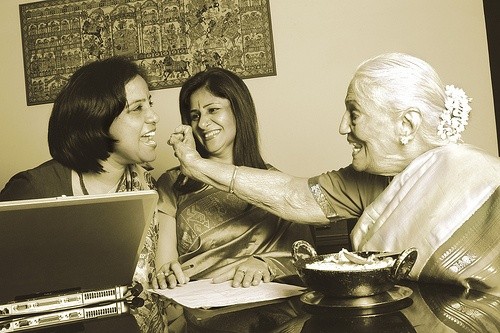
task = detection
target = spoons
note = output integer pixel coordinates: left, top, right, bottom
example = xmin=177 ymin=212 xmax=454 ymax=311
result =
xmin=342 ymin=245 xmax=401 ymax=264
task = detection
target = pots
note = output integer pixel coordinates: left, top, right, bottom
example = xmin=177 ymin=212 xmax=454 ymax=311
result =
xmin=292 ymin=238 xmax=418 ymax=295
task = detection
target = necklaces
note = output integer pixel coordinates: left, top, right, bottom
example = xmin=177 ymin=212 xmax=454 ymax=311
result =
xmin=78 ymin=169 xmax=135 ymax=195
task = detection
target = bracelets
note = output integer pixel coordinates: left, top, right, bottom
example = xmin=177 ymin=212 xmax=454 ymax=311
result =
xmin=229 ymin=166 xmax=238 ymax=194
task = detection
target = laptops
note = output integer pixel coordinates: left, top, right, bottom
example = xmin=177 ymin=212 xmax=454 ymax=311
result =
xmin=0 ymin=190 xmax=159 ymax=317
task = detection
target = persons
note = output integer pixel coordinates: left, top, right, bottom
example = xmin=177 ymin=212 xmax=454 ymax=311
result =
xmin=0 ymin=56 xmax=167 ymax=332
xmin=163 ymin=295 xmax=312 ymax=333
xmin=167 ymin=52 xmax=500 ymax=332
xmin=151 ymin=67 xmax=318 ymax=292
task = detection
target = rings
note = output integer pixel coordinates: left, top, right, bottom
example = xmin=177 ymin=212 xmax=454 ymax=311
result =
xmin=237 ymin=269 xmax=246 ymax=275
xmin=156 ymin=272 xmax=164 ymax=278
